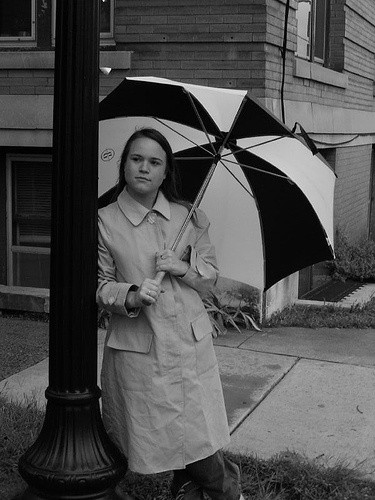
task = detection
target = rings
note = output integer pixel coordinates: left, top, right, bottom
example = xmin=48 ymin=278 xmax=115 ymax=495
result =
xmin=160 ymin=255 xmax=164 ymax=259
xmin=147 ymin=290 xmax=152 ymax=295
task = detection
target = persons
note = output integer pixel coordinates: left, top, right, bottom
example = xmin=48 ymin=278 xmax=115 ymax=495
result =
xmin=96 ymin=129 xmax=245 ymax=500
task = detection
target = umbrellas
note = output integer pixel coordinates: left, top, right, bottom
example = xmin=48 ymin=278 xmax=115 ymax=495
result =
xmin=98 ymin=76 xmax=338 ymax=306
